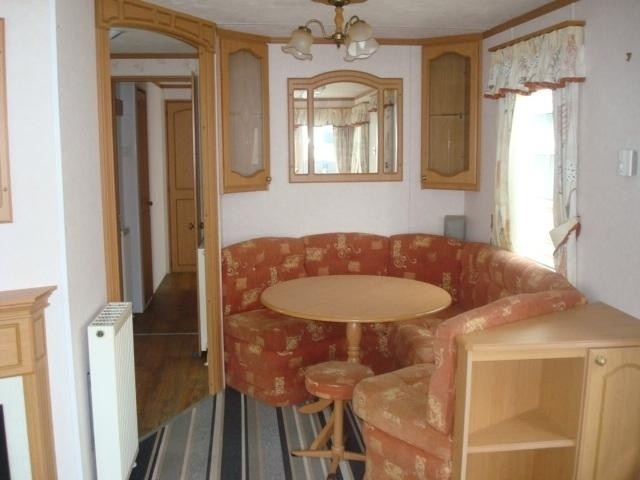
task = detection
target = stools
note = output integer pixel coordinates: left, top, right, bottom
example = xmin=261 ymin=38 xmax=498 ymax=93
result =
xmin=289 ymin=360 xmax=374 ymax=479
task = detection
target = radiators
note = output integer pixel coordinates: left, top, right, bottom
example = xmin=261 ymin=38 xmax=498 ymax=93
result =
xmin=88 ymin=300 xmax=138 ymax=480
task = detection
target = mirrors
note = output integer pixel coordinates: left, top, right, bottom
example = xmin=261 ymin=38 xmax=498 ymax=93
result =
xmin=287 ymin=70 xmax=404 ymax=183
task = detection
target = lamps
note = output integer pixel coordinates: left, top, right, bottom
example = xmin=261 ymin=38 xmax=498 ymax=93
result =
xmin=284 ymin=1 xmax=379 ymax=64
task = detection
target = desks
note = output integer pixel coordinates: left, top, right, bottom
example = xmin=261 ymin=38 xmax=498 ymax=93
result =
xmin=259 ymin=273 xmax=451 ymax=413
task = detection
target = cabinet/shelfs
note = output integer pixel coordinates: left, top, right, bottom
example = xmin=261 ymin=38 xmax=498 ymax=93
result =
xmin=453 ymin=301 xmax=639 ymax=480
xmin=462 ymin=353 xmax=586 ymax=478
xmin=420 ymin=32 xmax=484 ymax=191
xmin=0 ymin=283 xmax=63 ymax=480
xmin=217 ymin=41 xmax=271 ymax=193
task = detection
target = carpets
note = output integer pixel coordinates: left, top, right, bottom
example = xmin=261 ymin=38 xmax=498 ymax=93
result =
xmin=127 ymin=388 xmax=369 ymax=480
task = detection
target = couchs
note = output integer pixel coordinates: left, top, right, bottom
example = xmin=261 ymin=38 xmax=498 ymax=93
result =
xmin=226 ymin=233 xmax=574 ymax=477
